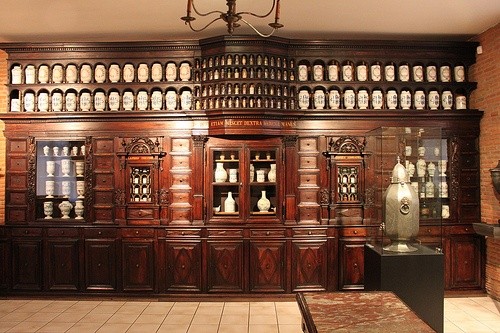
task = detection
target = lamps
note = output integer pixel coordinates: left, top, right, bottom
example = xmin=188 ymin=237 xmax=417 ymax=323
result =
xmin=487 ymin=159 xmax=500 ymax=223
xmin=181 ymin=0 xmax=284 ymax=39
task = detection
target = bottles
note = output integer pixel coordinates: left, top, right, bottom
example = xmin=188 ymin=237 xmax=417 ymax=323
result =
xmin=10 ymin=63 xmax=193 ymax=111
xmin=250 ymin=164 xmax=254 ymax=182
xmin=440 ymin=66 xmax=451 ymax=82
xmin=454 ymin=66 xmax=465 ymax=82
xmin=193 ymin=55 xmax=295 ymax=110
xmin=224 ymin=192 xmax=236 ymax=212
xmin=299 ymin=90 xmax=467 ymax=110
xmin=371 ymin=65 xmax=382 ymax=82
xmin=343 ymin=65 xmax=352 ymax=81
xmin=313 ymin=65 xmax=323 ymax=81
xmin=357 ymin=66 xmax=367 ymax=81
xmin=257 ymin=191 xmax=270 ymax=212
xmin=413 ymin=66 xmax=423 ymax=82
xmin=385 ymin=65 xmax=395 ymax=81
xmin=405 ymin=146 xmax=450 ymax=219
xmin=427 ymin=66 xmax=437 ymax=82
xmin=212 ymin=163 xmax=227 ymax=182
xmin=329 ymin=65 xmax=338 ymax=81
xmin=399 ymin=65 xmax=410 ymax=82
xmin=337 ymin=167 xmax=356 ymax=201
xmin=298 ymin=64 xmax=307 ymax=82
xmin=268 ymin=164 xmax=277 ymax=183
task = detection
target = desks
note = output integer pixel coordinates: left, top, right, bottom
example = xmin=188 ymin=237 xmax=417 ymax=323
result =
xmin=295 ymin=290 xmax=438 ymax=333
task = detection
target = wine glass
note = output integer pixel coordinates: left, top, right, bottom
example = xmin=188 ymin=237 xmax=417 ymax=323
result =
xmin=43 ymin=146 xmax=85 ymax=219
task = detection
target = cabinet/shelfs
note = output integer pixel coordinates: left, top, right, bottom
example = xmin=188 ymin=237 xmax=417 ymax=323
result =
xmin=418 ymin=225 xmax=486 ymax=294
xmin=8 ymin=226 xmax=83 ymax=298
xmin=83 ymin=225 xmax=157 ymax=297
xmin=401 ymin=126 xmax=456 ymax=223
xmin=25 ymin=135 xmax=96 ymax=225
xmin=334 ymin=228 xmax=383 ymax=293
xmin=317 ymin=135 xmax=376 ymax=225
xmin=248 ymin=224 xmax=334 ymax=299
xmin=204 ymin=135 xmax=284 ymax=224
xmin=4 ymin=50 xmax=195 ymax=113
xmin=157 ymin=223 xmax=250 ymax=298
xmin=294 ymin=56 xmax=478 ymax=110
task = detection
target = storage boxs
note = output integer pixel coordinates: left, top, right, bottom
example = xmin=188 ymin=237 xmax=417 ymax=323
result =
xmin=363 ymin=126 xmax=445 ymax=255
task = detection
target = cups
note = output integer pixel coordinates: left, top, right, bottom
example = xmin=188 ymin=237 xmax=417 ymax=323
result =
xmin=229 ymin=169 xmax=237 ymax=183
xmin=257 ymin=170 xmax=265 ymax=183
